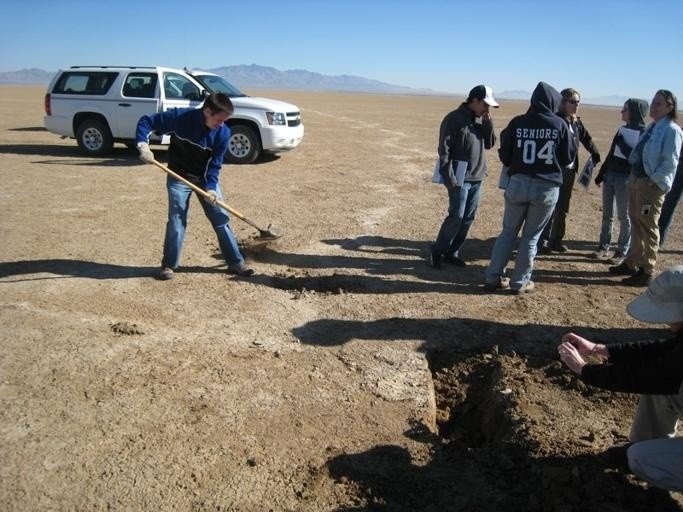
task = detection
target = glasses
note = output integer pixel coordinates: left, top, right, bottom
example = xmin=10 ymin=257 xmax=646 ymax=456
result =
xmin=567 ymin=100 xmax=579 ymax=104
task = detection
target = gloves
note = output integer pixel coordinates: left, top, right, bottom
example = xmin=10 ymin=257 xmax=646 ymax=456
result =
xmin=136 ymin=141 xmax=154 ymax=164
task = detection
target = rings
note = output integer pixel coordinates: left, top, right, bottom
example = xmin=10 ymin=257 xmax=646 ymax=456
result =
xmin=559 ymin=349 xmax=564 ymax=353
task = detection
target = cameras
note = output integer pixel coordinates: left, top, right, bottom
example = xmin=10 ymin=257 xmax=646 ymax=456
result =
xmin=557 ymin=340 xmax=579 ymax=349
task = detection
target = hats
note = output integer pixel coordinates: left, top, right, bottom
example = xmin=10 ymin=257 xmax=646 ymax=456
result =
xmin=470 ymin=85 xmax=500 ymax=108
xmin=626 ymin=266 xmax=682 ymax=324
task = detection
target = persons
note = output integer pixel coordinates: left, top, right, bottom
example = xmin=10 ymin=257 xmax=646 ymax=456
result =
xmin=135 ymin=93 xmax=254 ymax=280
xmin=591 ymin=99 xmax=649 ymax=267
xmin=608 ymin=90 xmax=682 ymax=285
xmin=484 ymin=81 xmax=577 ymax=294
xmin=658 ymin=143 xmax=683 ymax=246
xmin=557 ymin=265 xmax=682 ymax=490
xmin=430 ymin=86 xmax=499 ymax=269
xmin=536 ymin=88 xmax=600 ymax=255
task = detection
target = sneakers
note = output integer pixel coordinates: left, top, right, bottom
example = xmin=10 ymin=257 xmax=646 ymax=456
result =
xmin=431 ymin=242 xmax=652 ymax=294
xmin=236 ymin=264 xmax=254 ymax=277
xmin=607 ymin=441 xmax=631 ymax=463
xmin=160 ymin=266 xmax=173 ymax=278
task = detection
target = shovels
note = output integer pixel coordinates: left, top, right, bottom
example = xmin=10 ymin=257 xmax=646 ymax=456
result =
xmin=152 ymin=159 xmax=284 ymax=240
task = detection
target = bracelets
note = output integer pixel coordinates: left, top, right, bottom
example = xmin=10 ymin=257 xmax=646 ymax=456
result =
xmin=592 ymin=343 xmax=597 ymax=353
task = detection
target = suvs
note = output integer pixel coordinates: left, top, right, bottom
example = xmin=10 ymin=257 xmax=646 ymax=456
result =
xmin=42 ymin=66 xmax=306 ymax=164
